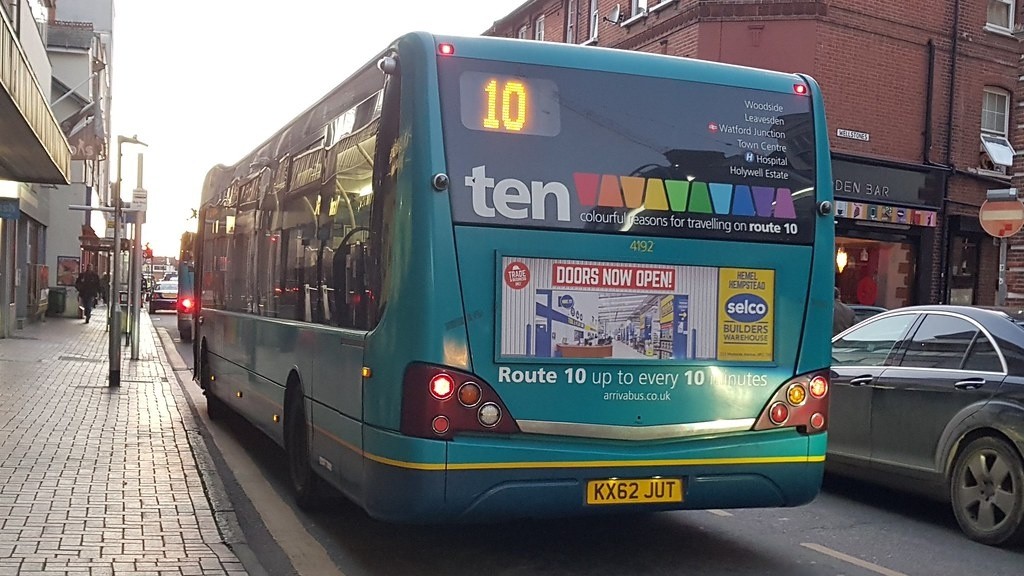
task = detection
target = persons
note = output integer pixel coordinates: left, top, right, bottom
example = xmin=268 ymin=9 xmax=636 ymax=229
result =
xmin=833 ymin=287 xmax=858 ymax=338
xmin=75 ymin=263 xmax=102 ymax=324
xmin=139 ymin=273 xmax=149 ymax=292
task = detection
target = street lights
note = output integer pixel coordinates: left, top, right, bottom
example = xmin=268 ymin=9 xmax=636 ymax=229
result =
xmin=107 ymin=134 xmax=148 ymax=386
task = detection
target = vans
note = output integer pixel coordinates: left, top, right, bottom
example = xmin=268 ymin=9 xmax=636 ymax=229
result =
xmin=143 ymin=274 xmax=156 ymax=292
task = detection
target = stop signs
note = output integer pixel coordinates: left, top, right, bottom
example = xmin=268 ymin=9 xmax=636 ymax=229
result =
xmin=979 ymin=198 xmax=1024 ymax=237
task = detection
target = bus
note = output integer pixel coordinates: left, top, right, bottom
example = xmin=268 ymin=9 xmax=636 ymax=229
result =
xmin=191 ymin=28 xmax=834 ymax=521
xmin=178 ymin=232 xmax=197 ymax=342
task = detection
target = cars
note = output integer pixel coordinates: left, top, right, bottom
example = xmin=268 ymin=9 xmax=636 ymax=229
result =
xmin=149 ymin=282 xmax=177 ymax=314
xmin=828 ymin=304 xmax=1024 ymax=549
